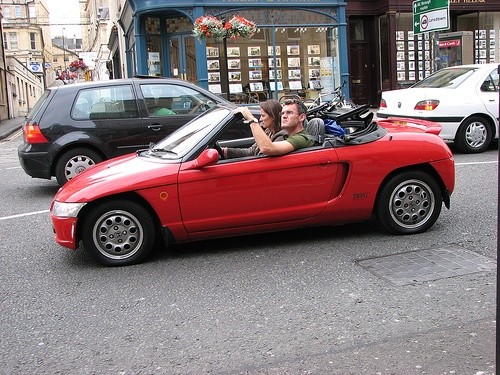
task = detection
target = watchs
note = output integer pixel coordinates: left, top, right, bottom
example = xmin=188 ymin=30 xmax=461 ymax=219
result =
xmin=249 ymin=117 xmax=259 ymax=124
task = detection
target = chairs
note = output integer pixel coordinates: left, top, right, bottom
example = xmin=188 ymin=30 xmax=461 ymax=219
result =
xmin=306 ymin=118 xmax=324 ymax=144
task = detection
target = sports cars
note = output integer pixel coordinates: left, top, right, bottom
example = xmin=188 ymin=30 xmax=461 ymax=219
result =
xmin=49 ymin=99 xmax=456 ymax=268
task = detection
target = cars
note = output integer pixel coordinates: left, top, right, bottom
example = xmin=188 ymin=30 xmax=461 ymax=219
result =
xmin=375 ymin=62 xmax=500 ymax=153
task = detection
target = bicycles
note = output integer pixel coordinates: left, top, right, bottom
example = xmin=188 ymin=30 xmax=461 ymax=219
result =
xmin=302 ymin=80 xmax=347 ymax=120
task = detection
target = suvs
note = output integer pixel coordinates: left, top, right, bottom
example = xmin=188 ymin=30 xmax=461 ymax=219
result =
xmin=18 ymin=73 xmax=266 ymax=188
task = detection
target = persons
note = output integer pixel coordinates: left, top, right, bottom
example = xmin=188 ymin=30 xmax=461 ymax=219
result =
xmin=221 ymin=99 xmax=288 ymax=159
xmin=234 ymin=99 xmax=315 ymax=156
xmin=153 ymin=93 xmax=204 ymax=116
